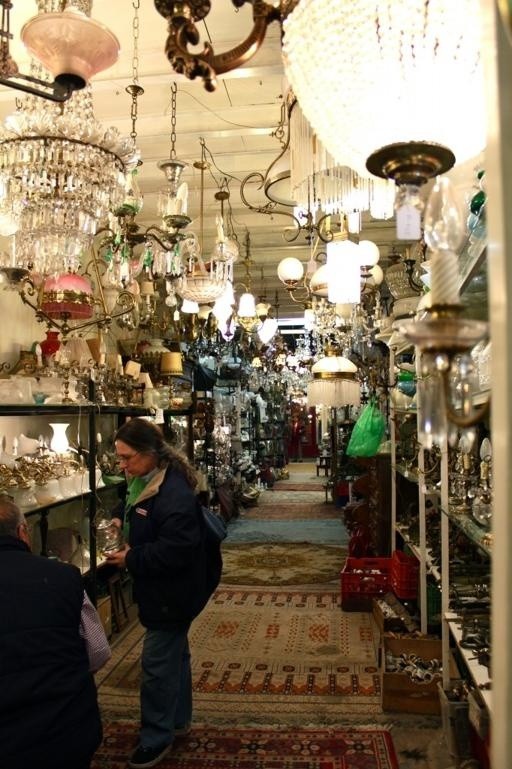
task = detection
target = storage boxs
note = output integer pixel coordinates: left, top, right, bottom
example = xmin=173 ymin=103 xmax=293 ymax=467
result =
xmin=380 ymin=637 xmax=463 ymax=717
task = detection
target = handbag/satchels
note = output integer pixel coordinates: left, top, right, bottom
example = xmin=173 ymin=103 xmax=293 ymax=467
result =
xmin=199 ymin=504 xmax=228 ymax=541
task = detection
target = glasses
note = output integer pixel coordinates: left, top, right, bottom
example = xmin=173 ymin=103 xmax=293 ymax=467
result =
xmin=117 ymin=452 xmax=140 ymax=462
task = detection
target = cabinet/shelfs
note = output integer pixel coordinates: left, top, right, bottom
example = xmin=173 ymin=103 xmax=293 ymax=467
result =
xmin=0 ymin=403 xmax=194 ymax=618
xmin=375 ymin=2 xmax=510 ymax=769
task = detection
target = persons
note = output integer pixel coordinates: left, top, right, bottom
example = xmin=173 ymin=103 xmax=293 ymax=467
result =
xmin=0 ymin=491 xmax=112 ymax=768
xmin=103 ymin=418 xmax=208 ymax=768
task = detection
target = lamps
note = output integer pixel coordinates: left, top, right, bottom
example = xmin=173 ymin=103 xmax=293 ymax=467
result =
xmin=0 ymin=1 xmax=511 ymax=566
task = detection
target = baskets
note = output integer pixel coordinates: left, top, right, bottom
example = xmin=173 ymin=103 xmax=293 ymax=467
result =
xmin=389 ymin=546 xmax=441 ymax=615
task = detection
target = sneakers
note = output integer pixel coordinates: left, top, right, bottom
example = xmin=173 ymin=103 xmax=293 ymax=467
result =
xmin=128 ymin=724 xmax=193 ymax=767
